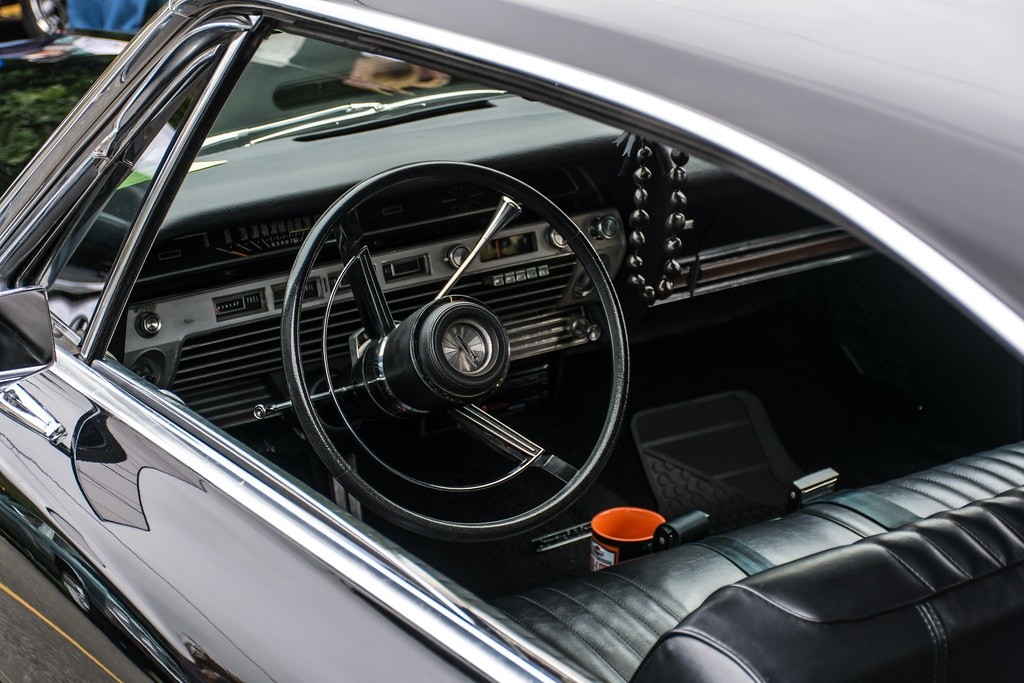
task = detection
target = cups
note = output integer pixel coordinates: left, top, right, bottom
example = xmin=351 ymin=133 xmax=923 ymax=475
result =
xmin=590 ymin=506 xmax=665 ymax=572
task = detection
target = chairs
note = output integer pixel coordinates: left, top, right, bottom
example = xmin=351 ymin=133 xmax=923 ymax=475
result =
xmin=496 ymin=435 xmax=1024 ymax=683
